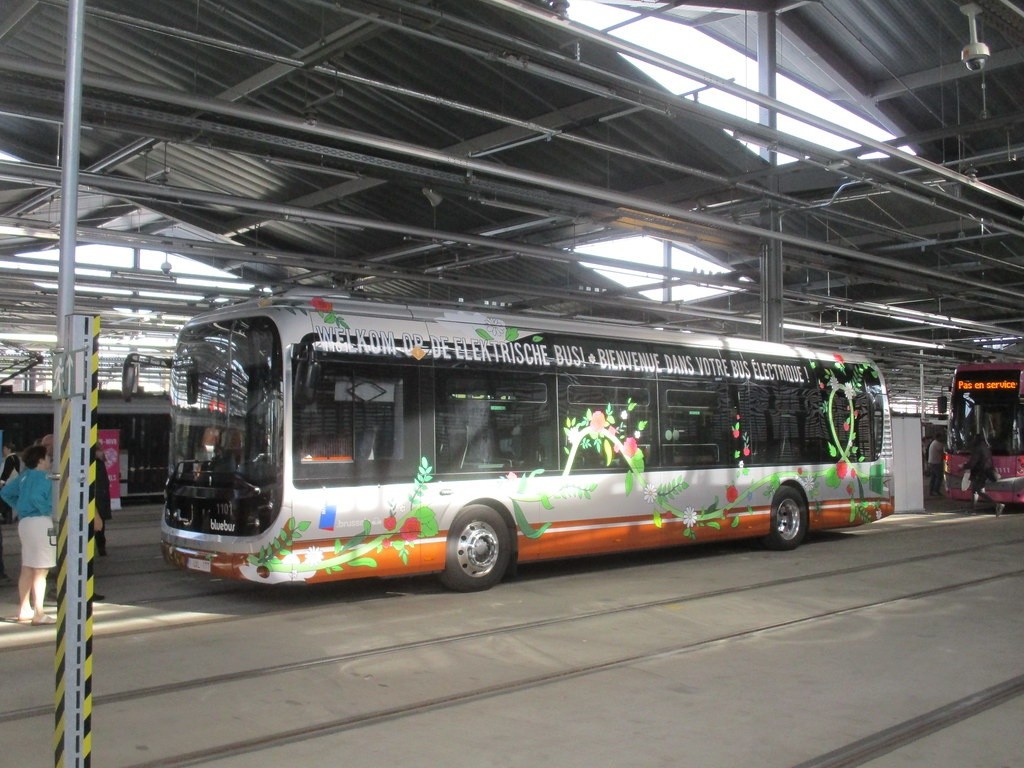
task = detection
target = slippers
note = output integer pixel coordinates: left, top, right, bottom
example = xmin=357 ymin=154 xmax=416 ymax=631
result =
xmin=16 ymin=614 xmax=57 ymax=625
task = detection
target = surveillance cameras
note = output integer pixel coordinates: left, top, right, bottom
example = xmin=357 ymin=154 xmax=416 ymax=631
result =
xmin=161 ymin=262 xmax=172 ymax=275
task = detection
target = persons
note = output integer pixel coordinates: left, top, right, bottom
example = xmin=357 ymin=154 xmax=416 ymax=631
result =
xmin=957 ymin=433 xmax=1005 ymax=519
xmin=0 ymin=434 xmax=113 ymax=625
xmin=181 ymin=427 xmax=234 ymax=532
xmin=921 ymin=434 xmax=944 ymax=496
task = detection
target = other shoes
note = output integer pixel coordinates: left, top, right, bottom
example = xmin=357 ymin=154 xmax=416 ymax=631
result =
xmin=92 ymin=592 xmax=105 ymax=602
xmin=0 ymin=573 xmax=8 ymax=580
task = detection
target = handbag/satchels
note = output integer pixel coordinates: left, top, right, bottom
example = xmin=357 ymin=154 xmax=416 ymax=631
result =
xmin=6 ymin=455 xmax=18 ymax=483
xmin=94 ymin=505 xmax=103 ymax=532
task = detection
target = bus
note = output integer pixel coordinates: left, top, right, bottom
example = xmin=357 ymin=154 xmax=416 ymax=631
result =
xmin=0 ymin=393 xmax=171 ymax=506
xmin=938 ymin=363 xmax=1024 ymax=504
xmin=123 ymin=301 xmax=894 ymax=593
